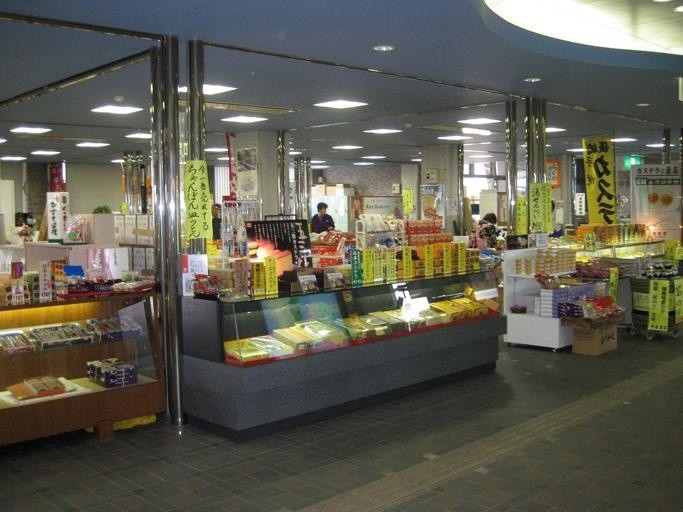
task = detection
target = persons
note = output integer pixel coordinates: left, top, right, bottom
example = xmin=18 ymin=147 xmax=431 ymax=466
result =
xmin=11 ymin=214 xmax=27 ymax=245
xmin=210 ymin=203 xmax=222 ymax=240
xmin=468 ymin=212 xmax=496 ymax=249
xmin=311 ymin=202 xmax=335 ymax=234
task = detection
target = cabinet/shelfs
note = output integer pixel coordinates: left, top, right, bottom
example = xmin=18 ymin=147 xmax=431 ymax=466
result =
xmin=575 ymin=238 xmax=668 ymax=327
xmin=0 ymin=291 xmax=169 ymax=446
xmin=24 ymin=213 xmax=114 ymax=271
xmin=182 ymin=268 xmax=508 ymax=443
xmin=503 ymin=246 xmax=630 ymax=352
xmin=311 ymin=195 xmax=362 ymax=236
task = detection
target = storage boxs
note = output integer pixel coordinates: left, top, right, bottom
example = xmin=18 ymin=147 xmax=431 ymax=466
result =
xmin=310 ymin=182 xmax=355 ymax=196
xmin=572 ymin=323 xmax=617 ymax=356
